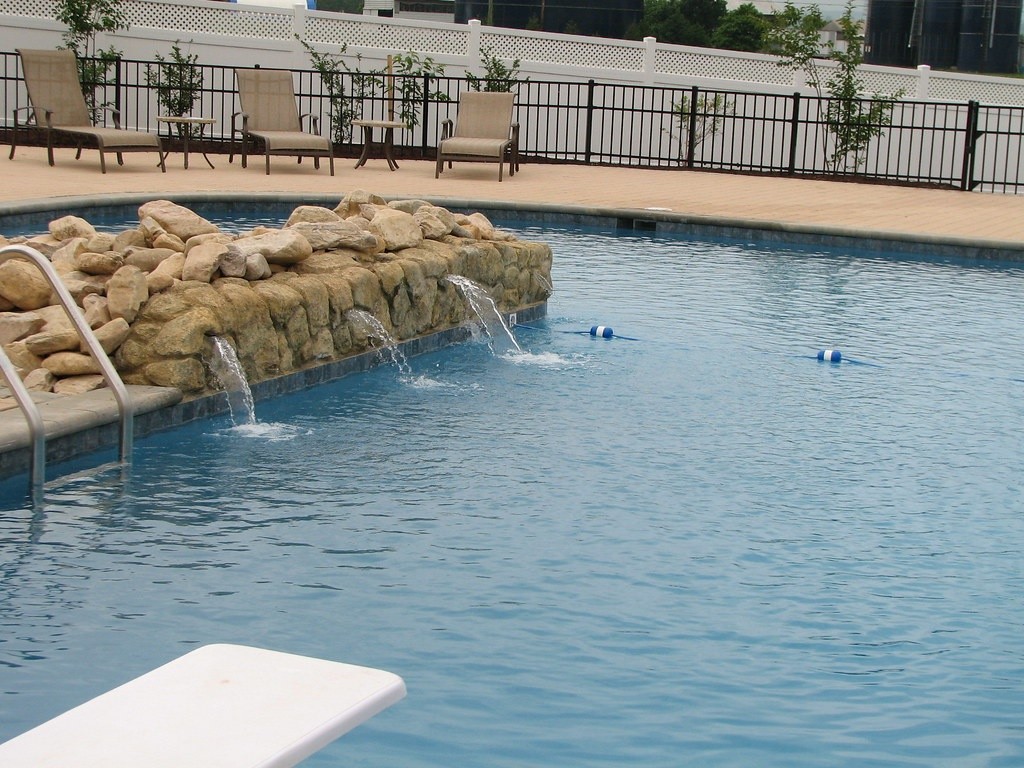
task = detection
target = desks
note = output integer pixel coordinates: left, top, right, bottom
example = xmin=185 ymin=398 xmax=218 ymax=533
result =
xmin=156 ymin=116 xmax=217 ymax=169
xmin=351 ymin=119 xmax=407 ymax=171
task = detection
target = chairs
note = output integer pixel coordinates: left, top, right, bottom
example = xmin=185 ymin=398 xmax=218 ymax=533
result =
xmin=8 ymin=49 xmax=167 ymax=175
xmin=228 ymin=68 xmax=334 ymax=176
xmin=435 ymin=90 xmax=520 ymax=182
